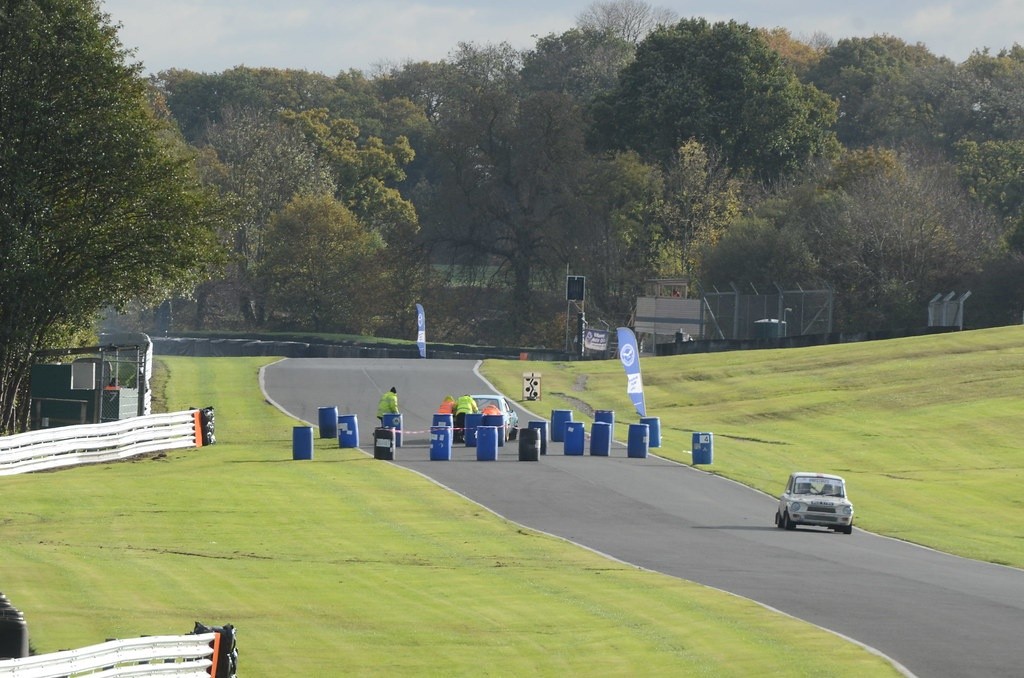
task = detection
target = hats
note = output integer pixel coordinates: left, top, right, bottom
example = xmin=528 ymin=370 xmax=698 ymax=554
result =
xmin=390 ymin=386 xmax=397 ymax=393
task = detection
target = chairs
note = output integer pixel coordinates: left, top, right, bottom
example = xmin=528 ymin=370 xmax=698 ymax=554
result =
xmin=821 ymin=484 xmax=834 ymax=494
xmin=796 ymin=482 xmax=810 ymax=494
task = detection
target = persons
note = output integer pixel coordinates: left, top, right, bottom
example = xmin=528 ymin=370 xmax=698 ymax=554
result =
xmin=670 ymin=289 xmax=681 ymax=297
xmin=103 ymin=376 xmax=122 ymax=389
xmin=377 ymin=387 xmax=399 ymax=424
xmin=453 ymin=394 xmax=479 ymax=443
xmin=437 ymin=395 xmax=455 ymax=414
xmin=675 ymin=328 xmax=683 ymax=355
xmin=481 ymin=404 xmax=501 ymax=415
xmin=798 ymin=485 xmax=810 ymax=494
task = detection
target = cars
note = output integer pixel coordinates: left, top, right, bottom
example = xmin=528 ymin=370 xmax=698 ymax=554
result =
xmin=775 ymin=472 xmax=853 ymax=534
xmin=470 ymin=395 xmax=519 ymax=442
